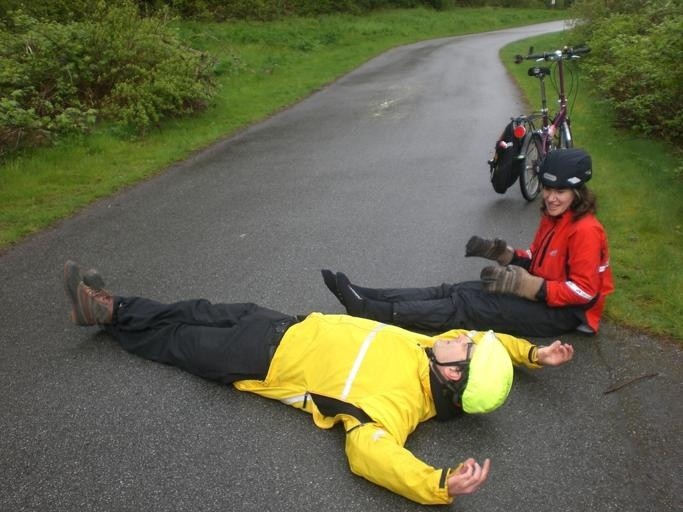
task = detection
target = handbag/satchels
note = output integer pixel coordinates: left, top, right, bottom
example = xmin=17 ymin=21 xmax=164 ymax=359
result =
xmin=488 ymin=123 xmax=523 ymax=193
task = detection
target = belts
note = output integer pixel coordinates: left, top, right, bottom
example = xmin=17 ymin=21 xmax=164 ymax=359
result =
xmin=268 ymin=316 xmax=294 ymax=365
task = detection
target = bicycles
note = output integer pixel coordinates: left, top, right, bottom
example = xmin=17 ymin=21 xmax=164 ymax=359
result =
xmin=513 ymin=44 xmax=592 ymax=202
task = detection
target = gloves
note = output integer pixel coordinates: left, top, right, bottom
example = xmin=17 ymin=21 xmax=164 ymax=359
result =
xmin=480 ymin=264 xmax=544 ymax=302
xmin=464 ymin=235 xmax=515 ymax=267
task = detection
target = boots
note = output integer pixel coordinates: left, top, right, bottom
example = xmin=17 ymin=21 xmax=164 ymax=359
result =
xmin=64 ymin=260 xmax=114 ymax=332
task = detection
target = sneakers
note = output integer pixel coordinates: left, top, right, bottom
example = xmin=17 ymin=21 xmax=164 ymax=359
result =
xmin=321 ymin=267 xmax=369 ymax=318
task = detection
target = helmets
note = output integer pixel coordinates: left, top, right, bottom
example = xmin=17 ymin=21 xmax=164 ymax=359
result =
xmin=540 ymin=147 xmax=592 ymax=188
xmin=462 ymin=329 xmax=514 ymax=415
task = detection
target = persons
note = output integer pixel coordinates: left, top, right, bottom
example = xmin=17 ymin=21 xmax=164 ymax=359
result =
xmin=318 ymin=147 xmax=615 ymax=339
xmin=60 ymin=258 xmax=575 ymax=503
